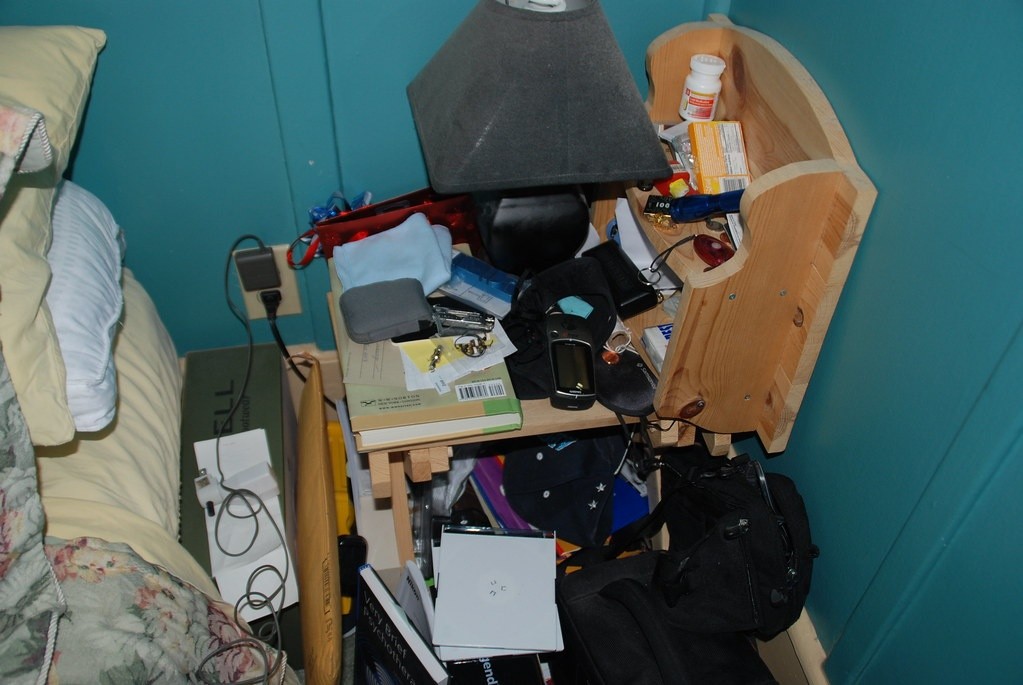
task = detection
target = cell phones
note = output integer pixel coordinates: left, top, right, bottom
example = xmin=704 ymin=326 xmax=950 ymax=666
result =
xmin=544 ymin=314 xmax=598 ymax=410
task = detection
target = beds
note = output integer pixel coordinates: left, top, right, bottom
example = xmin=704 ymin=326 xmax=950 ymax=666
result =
xmin=0 ymin=273 xmax=298 ymax=685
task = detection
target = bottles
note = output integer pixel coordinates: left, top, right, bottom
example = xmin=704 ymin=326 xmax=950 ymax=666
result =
xmin=678 ymin=53 xmax=727 ymax=124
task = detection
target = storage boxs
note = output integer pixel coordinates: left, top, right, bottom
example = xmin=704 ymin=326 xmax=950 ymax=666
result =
xmin=685 ymin=119 xmax=750 ymax=196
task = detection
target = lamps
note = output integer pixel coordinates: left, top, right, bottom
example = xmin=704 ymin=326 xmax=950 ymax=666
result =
xmin=280 ymin=16 xmax=875 ymax=608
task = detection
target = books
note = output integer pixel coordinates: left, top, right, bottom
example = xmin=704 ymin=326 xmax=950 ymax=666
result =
xmin=327 ymin=243 xmax=523 ymax=454
xmin=353 ymin=522 xmax=564 ymax=685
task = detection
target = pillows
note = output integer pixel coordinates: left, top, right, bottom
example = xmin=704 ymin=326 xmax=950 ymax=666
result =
xmin=0 ymin=22 xmax=105 ymax=448
xmin=39 ymin=175 xmax=125 ymax=431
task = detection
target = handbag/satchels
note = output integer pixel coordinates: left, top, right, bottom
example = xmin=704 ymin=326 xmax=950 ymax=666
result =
xmin=501 ymin=258 xmax=618 ymax=400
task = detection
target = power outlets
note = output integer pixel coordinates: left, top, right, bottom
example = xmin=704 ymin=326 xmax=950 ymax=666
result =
xmin=231 ymin=244 xmax=301 ymax=321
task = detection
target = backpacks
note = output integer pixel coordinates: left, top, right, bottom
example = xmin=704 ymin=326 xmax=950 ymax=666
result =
xmin=558 ymin=449 xmax=820 ymax=643
